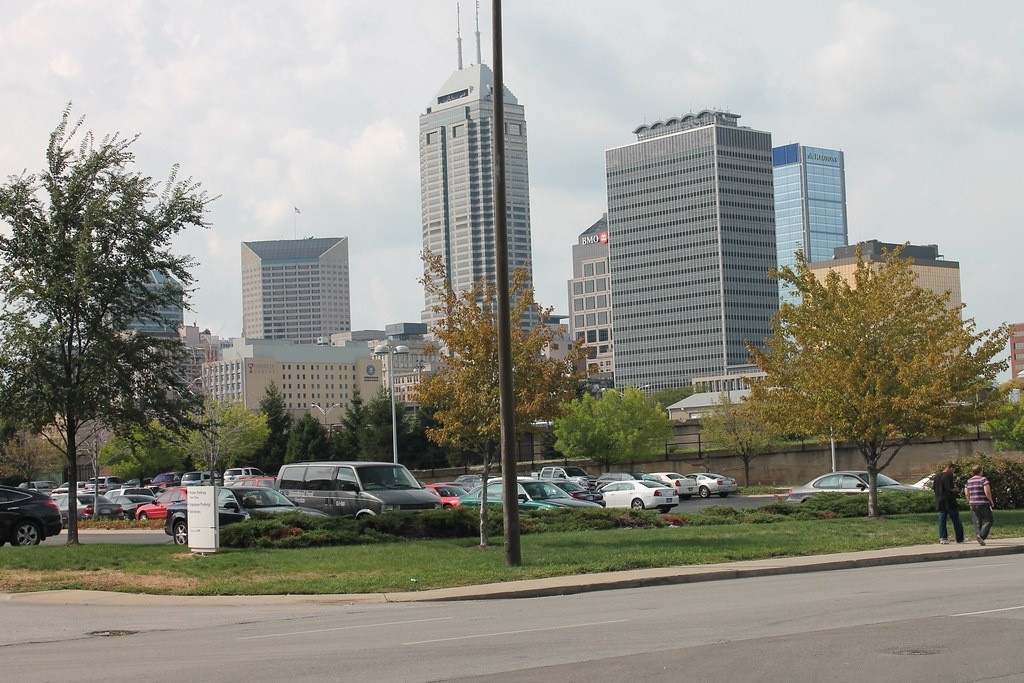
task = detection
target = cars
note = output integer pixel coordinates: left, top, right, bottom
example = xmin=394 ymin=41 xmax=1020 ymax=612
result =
xmin=597 ymin=479 xmax=680 ymax=514
xmin=786 ymin=470 xmax=919 ymax=508
xmin=684 ymin=472 xmax=738 ymax=498
xmin=913 ymin=472 xmax=967 ymax=498
xmin=0 ymin=484 xmax=64 ymax=547
xmin=17 ymin=466 xmax=278 ymax=522
xmin=647 ymin=472 xmax=699 ymax=500
xmin=420 ymin=464 xmax=672 ymax=506
xmin=163 ymin=486 xmax=331 ymax=547
xmin=457 ymin=477 xmax=607 ymax=517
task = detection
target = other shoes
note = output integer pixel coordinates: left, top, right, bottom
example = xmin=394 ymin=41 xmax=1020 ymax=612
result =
xmin=976 ymin=535 xmax=987 ymax=547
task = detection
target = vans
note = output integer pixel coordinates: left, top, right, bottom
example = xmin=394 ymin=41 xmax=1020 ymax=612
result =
xmin=272 ymin=460 xmax=442 ymax=521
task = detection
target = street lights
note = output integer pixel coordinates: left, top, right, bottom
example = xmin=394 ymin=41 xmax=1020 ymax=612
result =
xmin=310 ymin=403 xmax=340 ymax=438
xmin=374 ymin=344 xmax=411 ymax=483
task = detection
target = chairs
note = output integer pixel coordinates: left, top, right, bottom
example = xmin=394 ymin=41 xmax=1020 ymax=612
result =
xmin=245 ymin=496 xmax=257 ymax=507
xmin=527 ymin=488 xmax=536 ymax=500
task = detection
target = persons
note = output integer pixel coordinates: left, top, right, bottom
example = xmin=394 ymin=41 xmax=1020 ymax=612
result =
xmin=932 ymin=463 xmax=968 ymax=543
xmin=965 ymin=465 xmax=994 ymax=546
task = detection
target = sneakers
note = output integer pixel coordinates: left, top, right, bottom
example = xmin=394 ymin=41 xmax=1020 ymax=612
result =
xmin=956 ymin=537 xmax=968 ymax=544
xmin=940 ymin=540 xmax=950 ymax=544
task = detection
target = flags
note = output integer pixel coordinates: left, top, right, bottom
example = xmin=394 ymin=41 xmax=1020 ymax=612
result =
xmin=295 ymin=208 xmax=301 ymax=215
